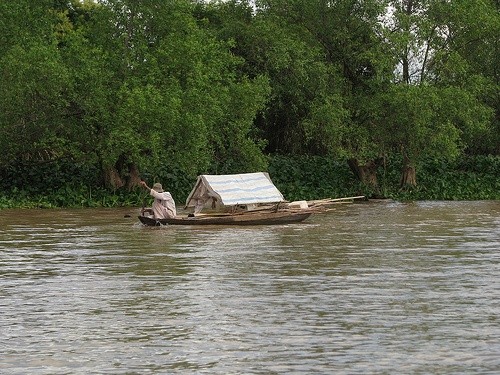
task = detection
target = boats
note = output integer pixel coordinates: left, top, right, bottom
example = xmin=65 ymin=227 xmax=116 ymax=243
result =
xmin=137 ymin=172 xmax=367 ymax=226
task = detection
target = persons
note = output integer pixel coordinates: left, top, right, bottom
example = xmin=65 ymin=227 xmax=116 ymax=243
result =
xmin=140 ymin=180 xmax=177 ymax=220
